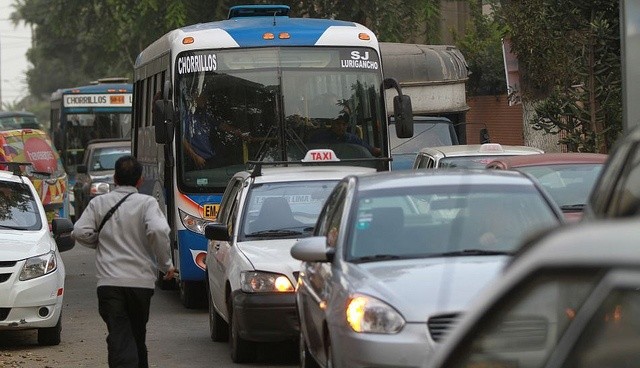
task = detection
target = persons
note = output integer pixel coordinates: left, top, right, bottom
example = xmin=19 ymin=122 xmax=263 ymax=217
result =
xmin=65 ymin=121 xmax=84 ymax=150
xmin=476 ymin=202 xmax=525 ymax=246
xmin=181 ymin=106 xmax=255 ymax=167
xmin=313 ymin=108 xmax=381 ymax=157
xmin=89 ymin=116 xmax=117 ymax=139
xmin=69 ymin=155 xmax=177 ymax=367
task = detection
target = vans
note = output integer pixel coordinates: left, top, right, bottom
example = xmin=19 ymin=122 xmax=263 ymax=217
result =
xmin=0 ymin=112 xmax=69 ymax=233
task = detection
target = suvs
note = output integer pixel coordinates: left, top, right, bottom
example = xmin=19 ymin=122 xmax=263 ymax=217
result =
xmin=585 ymin=127 xmax=640 ymax=217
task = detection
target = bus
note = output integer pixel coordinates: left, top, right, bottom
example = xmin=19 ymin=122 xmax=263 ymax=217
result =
xmin=50 ymin=79 xmax=134 ymax=217
xmin=206 ymin=159 xmax=393 ymax=362
xmin=133 ymin=6 xmax=412 ymax=311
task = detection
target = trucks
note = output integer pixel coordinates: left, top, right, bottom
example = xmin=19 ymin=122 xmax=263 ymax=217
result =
xmin=380 ymin=42 xmax=490 ymax=171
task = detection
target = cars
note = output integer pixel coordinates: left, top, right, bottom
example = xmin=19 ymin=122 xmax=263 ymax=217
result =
xmin=486 ymin=151 xmax=608 ymax=222
xmin=417 ymin=145 xmax=545 ymax=170
xmin=291 ymin=169 xmax=568 ymax=368
xmin=74 ymin=139 xmax=133 ymax=222
xmin=0 ymin=162 xmax=74 ymax=345
xmin=426 ymin=218 xmax=635 ymax=368
